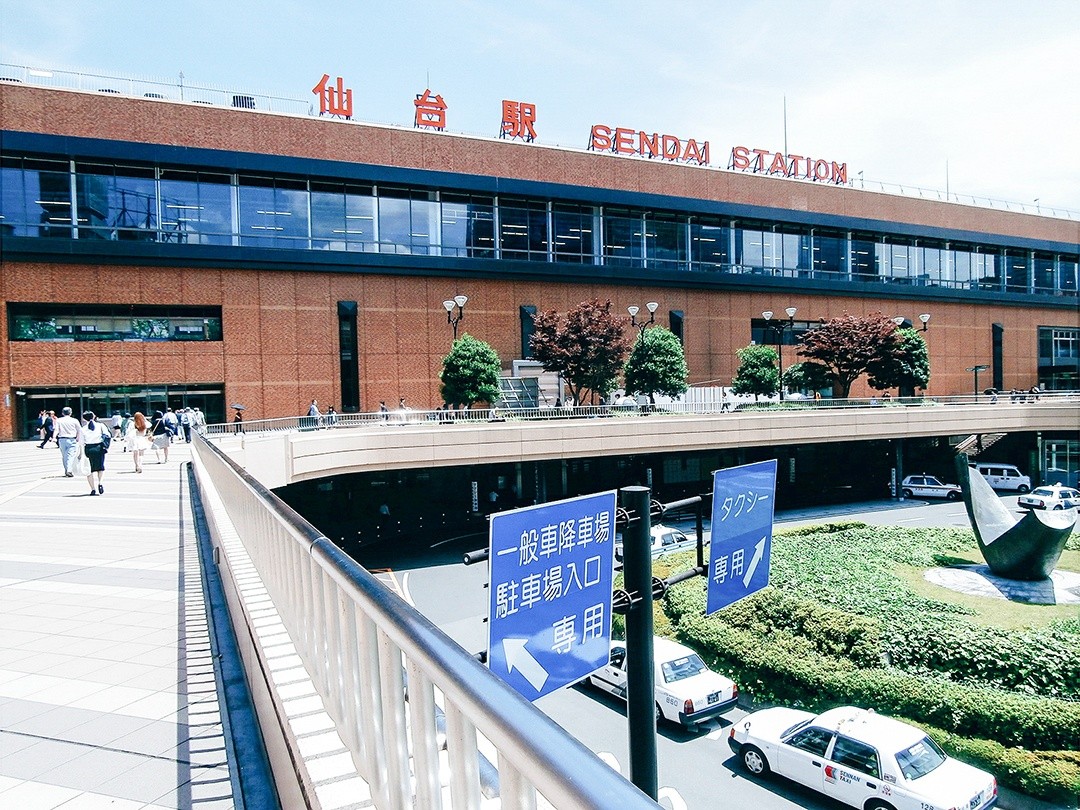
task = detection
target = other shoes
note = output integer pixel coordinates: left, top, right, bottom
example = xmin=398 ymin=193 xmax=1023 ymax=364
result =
xmin=158 ymin=462 xmax=161 ymax=464
xmin=136 ymin=466 xmax=138 ymax=471
xmin=165 ymin=459 xmax=168 ymax=462
xmin=179 ymin=438 xmax=181 ymax=440
xmin=90 ymin=490 xmax=96 ymax=496
xmin=36 ymin=446 xmax=43 ymax=450
xmin=65 ymin=472 xmax=73 ymax=477
xmin=129 ymin=450 xmax=132 ymax=452
xmin=113 ymin=439 xmax=116 ymax=441
xmin=98 ymin=484 xmax=104 ymax=494
xmin=182 ymin=437 xmax=184 ymax=439
xmin=171 ymin=441 xmax=173 ymax=443
xmin=123 ymin=447 xmax=126 ymax=452
xmin=139 ymin=469 xmax=142 ymax=473
xmin=121 ymin=439 xmax=124 ymax=441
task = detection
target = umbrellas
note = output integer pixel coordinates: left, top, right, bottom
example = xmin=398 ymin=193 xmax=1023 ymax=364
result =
xmin=984 ymin=388 xmax=998 ymax=395
xmin=231 ymin=404 xmax=245 ymax=416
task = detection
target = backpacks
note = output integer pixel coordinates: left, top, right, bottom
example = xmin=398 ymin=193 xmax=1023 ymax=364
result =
xmin=183 ymin=414 xmax=190 ymax=426
xmin=306 ymin=408 xmax=312 ymax=416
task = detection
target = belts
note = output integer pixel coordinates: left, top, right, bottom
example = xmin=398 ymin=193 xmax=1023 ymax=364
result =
xmin=60 ymin=437 xmax=76 ymax=439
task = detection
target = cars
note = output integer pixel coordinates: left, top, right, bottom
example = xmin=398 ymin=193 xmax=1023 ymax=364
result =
xmin=888 ymin=472 xmax=962 ymax=500
xmin=614 ymin=524 xmax=711 ymax=564
xmin=579 ymin=635 xmax=738 ymax=727
xmin=1017 ymin=486 xmax=1080 ymax=511
xmin=727 ymin=705 xmax=998 ymax=810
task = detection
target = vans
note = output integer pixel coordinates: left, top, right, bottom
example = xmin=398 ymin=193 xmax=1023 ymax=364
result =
xmin=968 ymin=461 xmax=1030 ymax=492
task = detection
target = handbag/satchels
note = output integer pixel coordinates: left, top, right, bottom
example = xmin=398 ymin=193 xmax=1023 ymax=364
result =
xmin=148 ymin=435 xmax=154 ymax=442
xmin=101 ymin=434 xmax=111 ymax=448
xmin=72 ymin=455 xmax=91 ymax=476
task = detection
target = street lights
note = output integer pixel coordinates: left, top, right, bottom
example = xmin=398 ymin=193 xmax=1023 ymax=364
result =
xmin=965 ymin=365 xmax=986 ymax=405
xmin=762 ymin=307 xmax=798 ymax=405
xmin=628 ymin=302 xmax=659 ymax=392
xmin=894 ymin=313 xmax=931 ymax=396
xmin=443 ymin=296 xmax=467 ymax=420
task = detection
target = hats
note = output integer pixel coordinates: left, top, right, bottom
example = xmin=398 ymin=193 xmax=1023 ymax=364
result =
xmin=113 ymin=410 xmax=120 ymax=414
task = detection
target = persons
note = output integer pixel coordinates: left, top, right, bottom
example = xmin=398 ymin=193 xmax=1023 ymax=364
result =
xmin=52 ymin=407 xmax=82 ymax=478
xmin=77 ymin=411 xmax=111 ymax=496
xmin=553 ymin=394 xmax=623 ymax=417
xmin=124 ymin=412 xmax=152 ymax=473
xmin=151 ymin=410 xmax=175 ymax=464
xmin=309 ymin=398 xmax=501 ymax=426
xmin=721 ymin=392 xmax=729 ymax=414
xmin=990 ymin=385 xmax=1040 ymax=406
xmin=233 ymin=409 xmax=245 ymax=437
xmin=869 ymin=391 xmax=892 ymax=408
xmin=35 ymin=406 xmax=207 ymax=449
